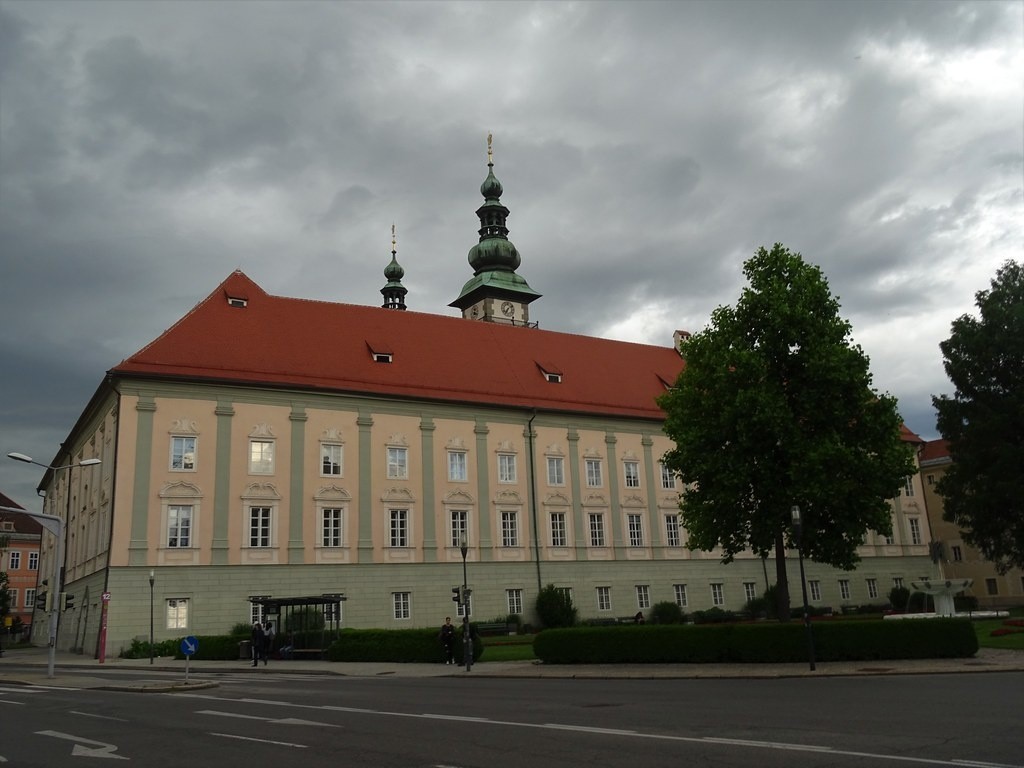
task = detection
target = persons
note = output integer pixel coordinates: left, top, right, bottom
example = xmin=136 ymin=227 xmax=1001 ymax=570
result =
xmin=457 ymin=617 xmax=475 ymax=666
xmin=279 ymin=629 xmax=294 ymax=660
xmin=442 ymin=616 xmax=455 ymax=665
xmin=634 ymin=612 xmax=644 ymax=625
xmin=249 ymin=621 xmax=274 ymax=667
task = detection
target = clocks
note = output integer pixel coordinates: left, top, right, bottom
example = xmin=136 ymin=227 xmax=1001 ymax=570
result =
xmin=501 ymin=302 xmax=515 ymax=316
xmin=471 ymin=305 xmax=478 ymax=320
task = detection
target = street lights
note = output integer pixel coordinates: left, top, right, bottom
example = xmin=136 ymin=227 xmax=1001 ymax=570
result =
xmin=790 ymin=505 xmax=815 ymax=671
xmin=461 ymin=541 xmax=473 ymax=671
xmin=149 ymin=570 xmax=156 ymax=664
xmin=8 ymin=452 xmax=103 ymax=680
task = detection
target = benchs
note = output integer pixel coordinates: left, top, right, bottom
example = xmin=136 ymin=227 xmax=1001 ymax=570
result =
xmin=288 ymin=647 xmax=327 ymax=659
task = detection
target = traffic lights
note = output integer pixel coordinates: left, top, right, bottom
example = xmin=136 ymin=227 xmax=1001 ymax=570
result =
xmin=452 ymin=584 xmax=465 ymax=605
xmin=38 ymin=577 xmax=55 ymax=613
xmin=61 ymin=593 xmax=75 ymax=612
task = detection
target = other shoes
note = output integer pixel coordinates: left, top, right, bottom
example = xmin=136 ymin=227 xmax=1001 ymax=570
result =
xmin=458 ymin=662 xmax=465 ymax=666
xmin=252 ymin=664 xmax=257 ymax=667
xmin=446 ymin=661 xmax=449 ymax=664
xmin=452 ymin=658 xmax=455 ymax=663
xmin=467 ymin=662 xmax=470 ymax=671
xmin=263 ymin=656 xmax=268 ymax=665
